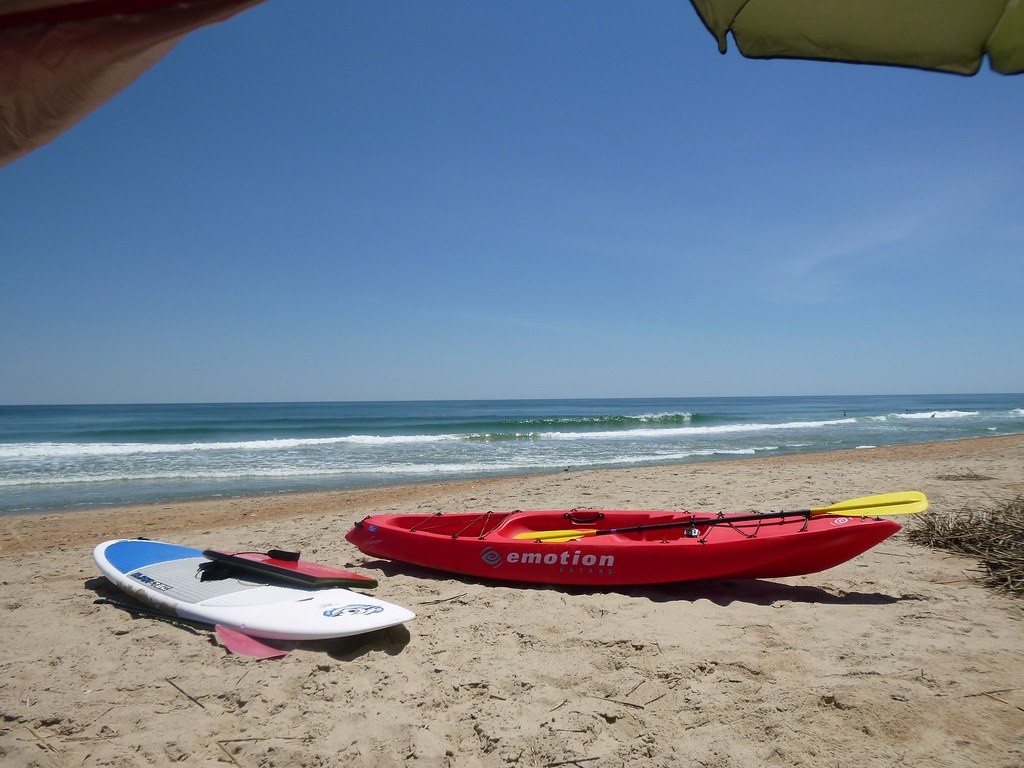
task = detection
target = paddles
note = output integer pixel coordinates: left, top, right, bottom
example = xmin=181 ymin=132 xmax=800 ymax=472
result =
xmin=512 ymin=490 xmax=929 ymax=544
xmin=92 ymin=598 xmax=289 ymax=661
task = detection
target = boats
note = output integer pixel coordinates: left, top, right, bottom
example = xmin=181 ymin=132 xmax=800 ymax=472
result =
xmin=344 ymin=506 xmax=904 ymax=589
